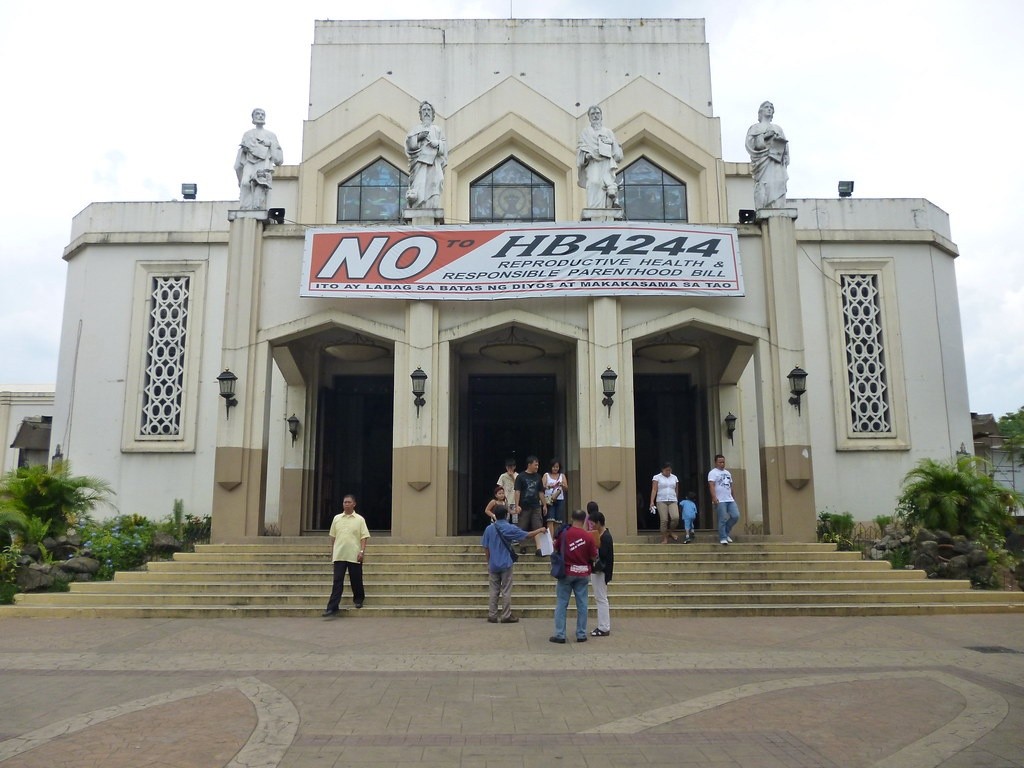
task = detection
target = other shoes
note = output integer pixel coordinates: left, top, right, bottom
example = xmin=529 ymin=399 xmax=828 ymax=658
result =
xmin=322 ymin=609 xmax=335 ymax=616
xmin=727 ymin=536 xmax=732 ymax=543
xmin=487 ymin=617 xmax=497 ymax=623
xmin=549 ymin=637 xmax=565 ymax=643
xmin=720 ymin=540 xmax=728 ymax=543
xmin=669 ymin=533 xmax=678 ymax=541
xmin=690 ymin=529 xmax=695 ymax=538
xmin=356 ymin=603 xmax=363 ymax=608
xmin=683 ymin=537 xmax=691 ymax=544
xmin=501 ymin=614 xmax=519 ymax=623
xmin=578 ymin=637 xmax=587 ymax=642
xmin=520 ymin=547 xmax=526 ymax=554
xmin=535 ymin=548 xmax=547 ymax=557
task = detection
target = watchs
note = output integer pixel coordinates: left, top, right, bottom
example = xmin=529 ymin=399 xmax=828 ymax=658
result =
xmin=360 ymin=550 xmax=364 ymax=554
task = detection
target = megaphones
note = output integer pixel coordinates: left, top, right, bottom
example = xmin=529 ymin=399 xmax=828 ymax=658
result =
xmin=738 ymin=209 xmax=755 ymax=224
xmin=269 ymin=208 xmax=285 ymax=225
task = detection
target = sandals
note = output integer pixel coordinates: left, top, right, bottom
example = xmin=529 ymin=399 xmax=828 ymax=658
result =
xmin=590 ymin=628 xmax=610 ymax=636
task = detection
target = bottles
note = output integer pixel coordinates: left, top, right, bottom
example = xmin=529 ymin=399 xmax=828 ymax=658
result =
xmin=551 ymin=489 xmax=561 ymax=500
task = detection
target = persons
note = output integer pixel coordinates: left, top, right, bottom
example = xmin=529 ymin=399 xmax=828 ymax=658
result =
xmin=577 ymin=105 xmax=624 ymax=208
xmin=679 ymin=491 xmax=698 ymax=543
xmin=484 ymin=455 xmax=568 ymax=557
xmin=583 ymin=501 xmax=614 ymax=636
xmin=479 ymin=504 xmax=548 ymax=623
xmin=234 ymin=108 xmax=283 ymax=210
xmin=708 ymin=455 xmax=740 ymax=543
xmin=322 ymin=494 xmax=370 ymax=616
xmin=745 ymin=101 xmax=790 ymax=210
xmin=404 ymin=101 xmax=448 ymax=208
xmin=650 ymin=461 xmax=679 ymax=544
xmin=549 ymin=509 xmax=598 ymax=643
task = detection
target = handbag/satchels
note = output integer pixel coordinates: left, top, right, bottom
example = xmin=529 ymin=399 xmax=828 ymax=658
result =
xmin=505 ymin=541 xmax=519 ymax=562
xmin=550 ymin=552 xmax=566 ymax=579
xmin=540 ymin=494 xmax=553 ymax=507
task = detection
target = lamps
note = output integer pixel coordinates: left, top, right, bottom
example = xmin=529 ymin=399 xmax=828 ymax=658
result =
xmin=601 ymin=365 xmax=618 ymax=419
xmin=786 ymin=364 xmax=809 ymax=418
xmin=838 ymin=181 xmax=856 ymax=198
xmin=725 ymin=411 xmax=737 ymax=447
xmin=635 ymin=333 xmax=701 ymax=364
xmin=738 ymin=209 xmax=755 ymax=224
xmin=479 ymin=326 xmax=547 ymax=366
xmin=268 ymin=208 xmax=286 ymax=224
xmin=181 ymin=183 xmax=197 ymax=200
xmin=410 ymin=364 xmax=428 ymax=418
xmin=217 ymin=367 xmax=238 ymax=419
xmin=286 ymin=413 xmax=299 ymax=448
xmin=324 ymin=332 xmax=390 ymax=362
